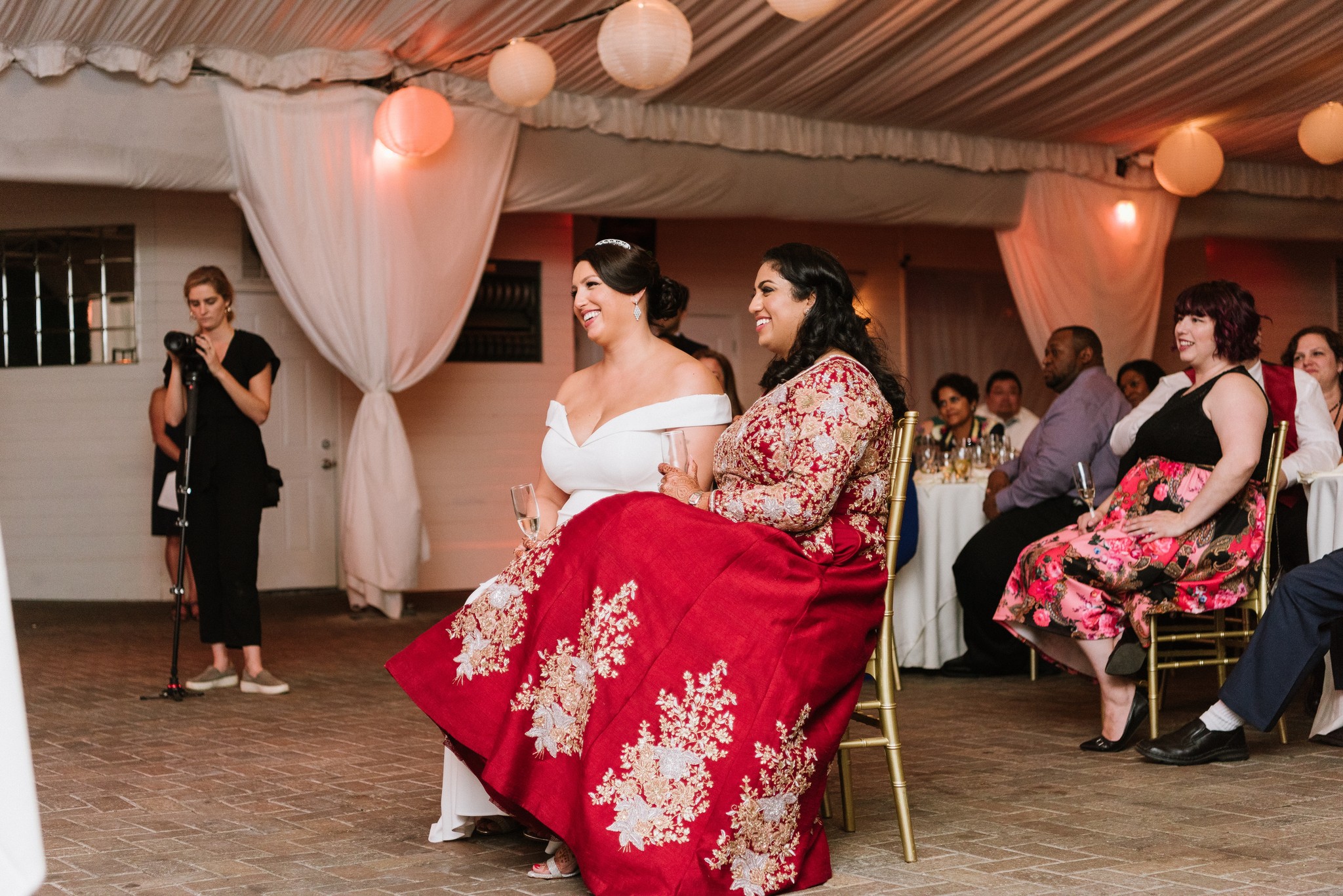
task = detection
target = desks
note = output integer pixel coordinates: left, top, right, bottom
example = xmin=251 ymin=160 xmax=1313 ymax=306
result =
xmin=892 ymin=478 xmax=988 ymax=669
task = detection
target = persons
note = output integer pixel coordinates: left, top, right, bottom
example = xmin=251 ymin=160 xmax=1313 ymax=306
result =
xmin=692 ymin=349 xmax=742 ymax=494
xmin=993 ymin=280 xmax=1274 ymax=755
xmin=1110 ymin=291 xmax=1343 ymax=716
xmin=383 ymin=243 xmax=906 ymax=896
xmin=1133 ymin=549 xmax=1343 ymax=766
xmin=974 ymin=368 xmax=1041 ymax=464
xmin=1117 ymin=359 xmax=1166 ymax=409
xmin=149 ymin=356 xmax=202 ymax=623
xmin=941 ymin=326 xmax=1135 ymax=678
xmin=165 ymin=265 xmax=291 ymax=695
xmin=917 ymin=372 xmax=1004 ymax=454
xmin=648 ymin=276 xmax=711 ymax=359
xmin=427 ymin=239 xmax=736 ymax=855
xmin=1279 ymin=326 xmax=1343 ymax=461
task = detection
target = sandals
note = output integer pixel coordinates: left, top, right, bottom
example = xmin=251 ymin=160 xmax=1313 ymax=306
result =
xmin=171 ymin=601 xmax=187 ymax=622
xmin=189 ymin=601 xmax=199 ymax=621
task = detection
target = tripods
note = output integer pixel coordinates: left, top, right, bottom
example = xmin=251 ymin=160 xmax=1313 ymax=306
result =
xmin=139 ymin=370 xmax=206 ymax=702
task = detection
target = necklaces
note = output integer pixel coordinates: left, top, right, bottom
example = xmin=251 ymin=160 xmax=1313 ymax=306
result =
xmin=1333 ymin=402 xmax=1343 ymax=424
xmin=1329 ymin=399 xmax=1343 ymax=413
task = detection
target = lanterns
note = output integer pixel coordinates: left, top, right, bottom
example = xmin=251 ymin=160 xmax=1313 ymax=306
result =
xmin=766 ymin=0 xmax=844 ymax=23
xmin=597 ymin=0 xmax=693 ymax=91
xmin=374 ymin=79 xmax=453 ymax=158
xmin=1154 ymin=126 xmax=1224 ymax=197
xmin=1297 ymin=102 xmax=1343 ymax=165
xmin=487 ymin=37 xmax=557 ymax=109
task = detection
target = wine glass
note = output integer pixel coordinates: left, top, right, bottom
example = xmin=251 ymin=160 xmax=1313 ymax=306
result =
xmin=510 ymin=483 xmax=541 ymax=543
xmin=1071 ymin=460 xmax=1100 ymax=531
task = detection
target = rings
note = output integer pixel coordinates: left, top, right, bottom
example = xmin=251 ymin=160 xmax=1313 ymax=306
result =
xmin=207 ymin=347 xmax=211 ymax=349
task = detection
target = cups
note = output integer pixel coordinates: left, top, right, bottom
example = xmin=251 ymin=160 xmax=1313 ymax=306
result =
xmin=915 ymin=432 xmax=1014 ymax=483
xmin=660 ymin=430 xmax=689 ymax=474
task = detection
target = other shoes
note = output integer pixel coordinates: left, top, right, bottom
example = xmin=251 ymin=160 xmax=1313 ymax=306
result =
xmin=186 ymin=662 xmax=238 ymax=690
xmin=240 ymin=667 xmax=289 ymax=695
xmin=939 ymin=656 xmax=990 ymax=678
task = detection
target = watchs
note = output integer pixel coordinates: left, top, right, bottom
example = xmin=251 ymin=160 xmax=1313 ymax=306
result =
xmin=688 ymin=491 xmax=705 ymax=506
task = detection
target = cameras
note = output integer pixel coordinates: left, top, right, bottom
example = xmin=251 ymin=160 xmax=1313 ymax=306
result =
xmin=163 ymin=332 xmax=207 ymax=369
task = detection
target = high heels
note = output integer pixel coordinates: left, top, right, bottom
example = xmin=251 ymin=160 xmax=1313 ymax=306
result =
xmin=523 ymin=829 xmax=562 ymax=855
xmin=1104 ymin=624 xmax=1146 ymax=677
xmin=475 ymin=813 xmax=520 ymax=832
xmin=1079 ymin=684 xmax=1148 ymax=751
xmin=527 ymin=843 xmax=580 ymax=879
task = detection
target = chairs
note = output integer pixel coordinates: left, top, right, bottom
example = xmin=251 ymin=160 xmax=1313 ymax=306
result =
xmin=824 ymin=412 xmax=918 ymax=863
xmin=1148 ymin=421 xmax=1288 ymax=746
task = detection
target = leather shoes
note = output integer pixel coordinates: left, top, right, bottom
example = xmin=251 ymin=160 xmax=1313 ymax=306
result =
xmin=1133 ymin=718 xmax=1249 ymax=765
